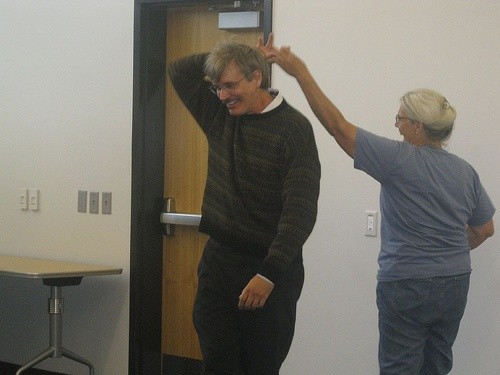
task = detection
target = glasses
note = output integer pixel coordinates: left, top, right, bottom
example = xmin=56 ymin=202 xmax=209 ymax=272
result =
xmin=395 ymin=114 xmax=419 ymax=124
xmin=209 ymin=68 xmax=261 ymax=96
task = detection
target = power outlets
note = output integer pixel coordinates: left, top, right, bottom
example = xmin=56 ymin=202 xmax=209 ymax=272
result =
xmin=90 ymin=191 xmax=98 ymax=214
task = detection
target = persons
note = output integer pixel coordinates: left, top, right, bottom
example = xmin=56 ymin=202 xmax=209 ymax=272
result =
xmin=272 ymin=46 xmax=496 ymax=375
xmin=168 ymin=33 xmax=321 ymax=375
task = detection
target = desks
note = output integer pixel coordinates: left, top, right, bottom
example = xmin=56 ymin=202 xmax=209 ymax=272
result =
xmin=0 ymin=256 xmax=123 ymax=375
